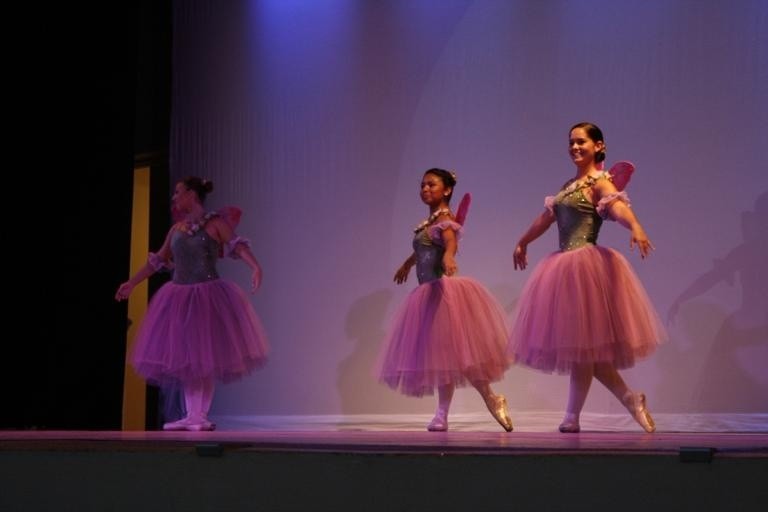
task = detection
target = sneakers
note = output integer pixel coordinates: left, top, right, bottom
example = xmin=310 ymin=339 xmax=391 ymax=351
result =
xmin=427 ymin=408 xmax=449 ymax=431
xmin=558 ymin=412 xmax=581 ymax=433
xmin=484 ymin=393 xmax=514 ymax=432
xmin=622 ymin=387 xmax=657 ymax=432
xmin=161 ymin=414 xmax=215 ymax=433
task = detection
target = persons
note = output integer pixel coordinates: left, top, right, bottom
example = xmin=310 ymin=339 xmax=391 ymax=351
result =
xmin=373 ymin=167 xmax=514 ymax=433
xmin=511 ymin=121 xmax=671 ymax=435
xmin=115 ymin=177 xmax=272 ymax=431
